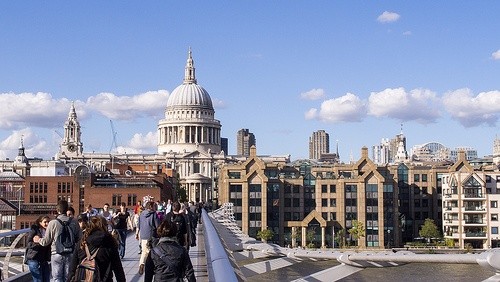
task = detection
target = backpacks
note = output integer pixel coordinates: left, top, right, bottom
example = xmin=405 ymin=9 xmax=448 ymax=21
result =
xmin=74 ymin=243 xmax=99 ymax=282
xmin=53 ymin=216 xmax=76 ymax=255
xmin=111 ymin=229 xmax=120 ymax=245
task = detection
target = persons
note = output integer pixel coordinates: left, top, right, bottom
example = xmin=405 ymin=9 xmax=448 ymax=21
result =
xmin=33 ymin=200 xmax=81 ymax=282
xmin=26 ymin=216 xmax=51 ymax=282
xmin=135 ymin=200 xmax=160 ymax=274
xmin=144 ymin=219 xmax=196 ymax=282
xmin=100 ymin=202 xmax=130 ymax=259
xmin=68 ymin=216 xmax=126 ymax=282
xmin=156 ymin=199 xmax=205 ymax=251
xmin=67 ymin=204 xmax=98 ymax=234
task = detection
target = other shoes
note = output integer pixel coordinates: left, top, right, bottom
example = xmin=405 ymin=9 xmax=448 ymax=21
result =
xmin=138 ymin=263 xmax=144 ymax=276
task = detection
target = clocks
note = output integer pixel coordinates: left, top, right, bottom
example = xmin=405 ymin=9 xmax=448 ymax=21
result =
xmin=67 ymin=144 xmax=77 ymax=152
